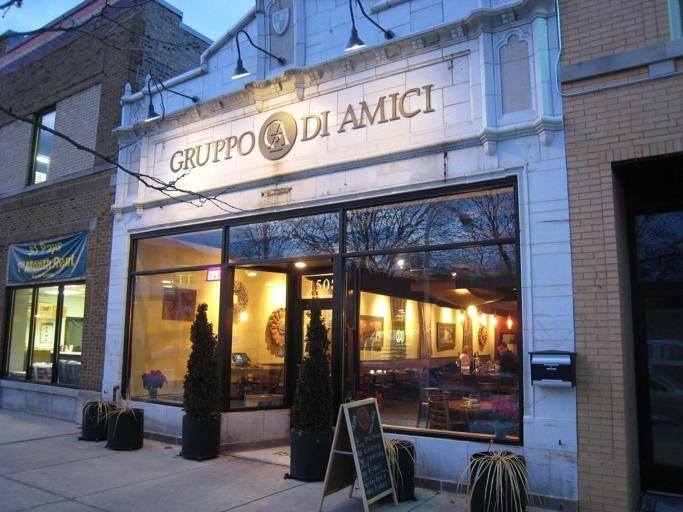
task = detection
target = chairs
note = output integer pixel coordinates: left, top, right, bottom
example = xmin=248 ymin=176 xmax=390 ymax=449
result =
xmin=415 ymin=369 xmax=519 ymax=430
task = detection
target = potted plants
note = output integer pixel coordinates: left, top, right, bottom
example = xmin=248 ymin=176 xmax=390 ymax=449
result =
xmin=181 ymin=301 xmax=223 ymax=461
xmin=290 ymin=294 xmax=336 ymax=481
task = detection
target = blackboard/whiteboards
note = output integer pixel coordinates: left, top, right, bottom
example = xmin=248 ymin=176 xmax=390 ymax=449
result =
xmin=343 ymin=398 xmax=395 ymax=505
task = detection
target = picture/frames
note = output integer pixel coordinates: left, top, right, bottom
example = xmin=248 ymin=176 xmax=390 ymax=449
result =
xmin=436 ymin=322 xmax=456 ymax=351
xmin=359 ymin=315 xmax=384 ymax=351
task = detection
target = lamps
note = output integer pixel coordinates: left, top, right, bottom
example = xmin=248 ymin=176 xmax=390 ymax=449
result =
xmin=344 ymin=1 xmax=396 ymax=53
xmin=230 ymin=29 xmax=287 ymax=81
xmin=143 ymin=76 xmax=201 ymax=124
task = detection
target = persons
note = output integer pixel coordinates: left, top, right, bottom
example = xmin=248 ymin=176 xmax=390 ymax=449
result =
xmin=493 ymin=341 xmax=519 ymax=387
xmin=454 ymin=345 xmax=481 ymax=370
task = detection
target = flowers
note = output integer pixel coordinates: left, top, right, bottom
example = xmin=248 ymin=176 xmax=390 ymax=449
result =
xmin=141 ymin=370 xmax=168 ymax=391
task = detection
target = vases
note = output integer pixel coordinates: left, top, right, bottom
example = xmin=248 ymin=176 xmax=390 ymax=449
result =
xmin=384 ymin=441 xmax=415 ymax=503
xmin=82 ymin=402 xmax=144 ymax=450
xmin=494 ymin=419 xmax=506 ymax=439
xmin=469 ymin=451 xmax=526 ymax=511
xmin=147 ymin=387 xmax=159 ymax=399
